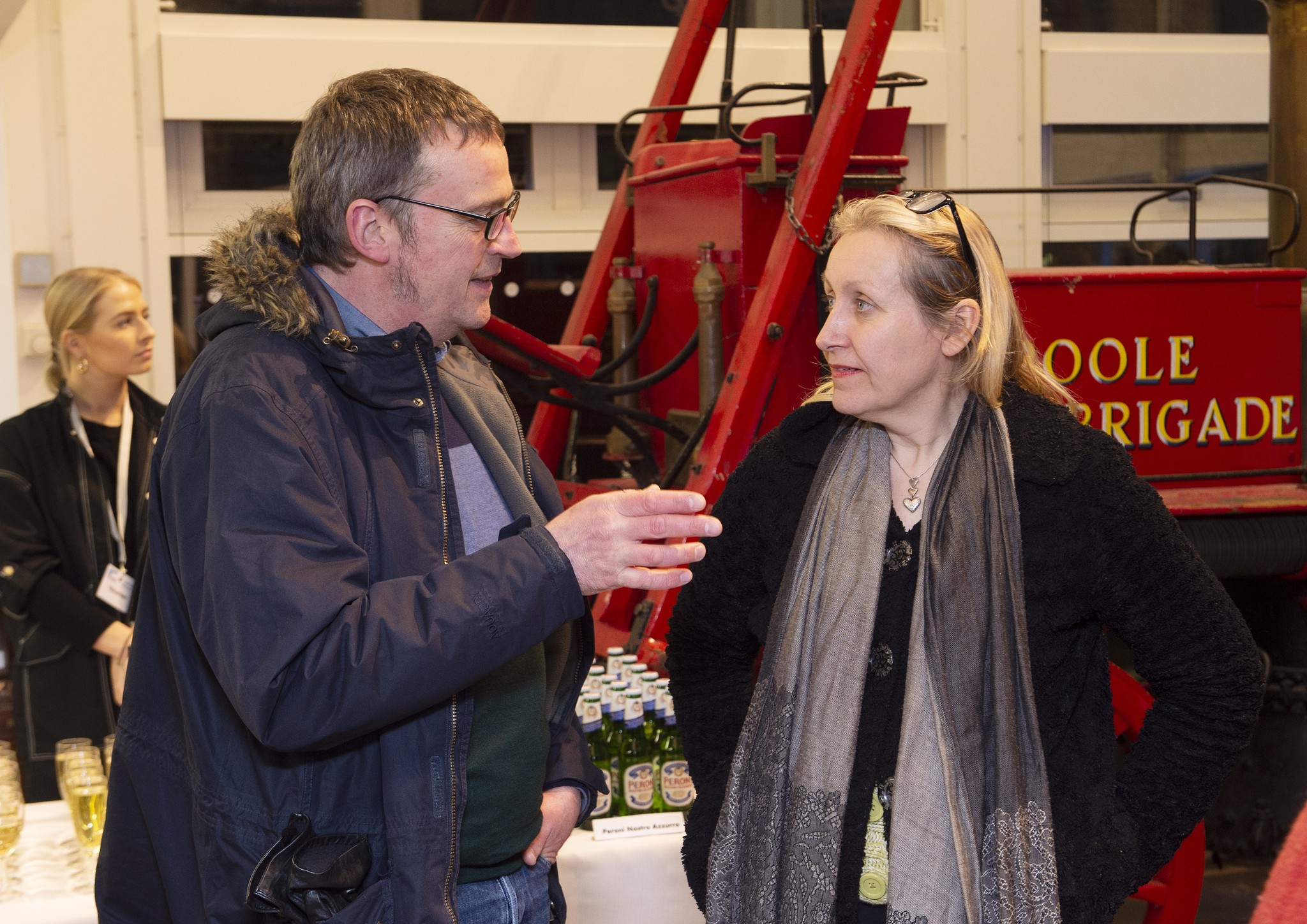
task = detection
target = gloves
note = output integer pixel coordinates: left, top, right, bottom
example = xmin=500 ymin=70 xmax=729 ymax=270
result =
xmin=241 ymin=812 xmax=375 ymax=924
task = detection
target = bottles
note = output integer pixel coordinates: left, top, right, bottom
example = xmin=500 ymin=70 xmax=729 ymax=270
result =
xmin=572 ymin=647 xmax=696 ymax=830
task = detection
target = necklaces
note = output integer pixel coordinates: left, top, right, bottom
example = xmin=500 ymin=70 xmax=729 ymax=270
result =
xmin=889 ymin=450 xmax=941 ymax=513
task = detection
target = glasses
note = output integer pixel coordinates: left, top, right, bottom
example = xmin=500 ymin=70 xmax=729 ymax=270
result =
xmin=894 ymin=189 xmax=985 ymax=302
xmin=370 ymin=189 xmax=522 ymax=241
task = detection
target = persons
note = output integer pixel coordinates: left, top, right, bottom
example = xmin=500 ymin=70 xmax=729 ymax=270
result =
xmin=1 ymin=267 xmax=167 ymax=804
xmin=665 ymin=192 xmax=1266 ymax=924
xmin=93 ymin=67 xmax=721 ymax=924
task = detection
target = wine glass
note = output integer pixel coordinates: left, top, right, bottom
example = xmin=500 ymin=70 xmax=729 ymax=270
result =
xmin=0 ymin=732 xmax=114 ymax=905
